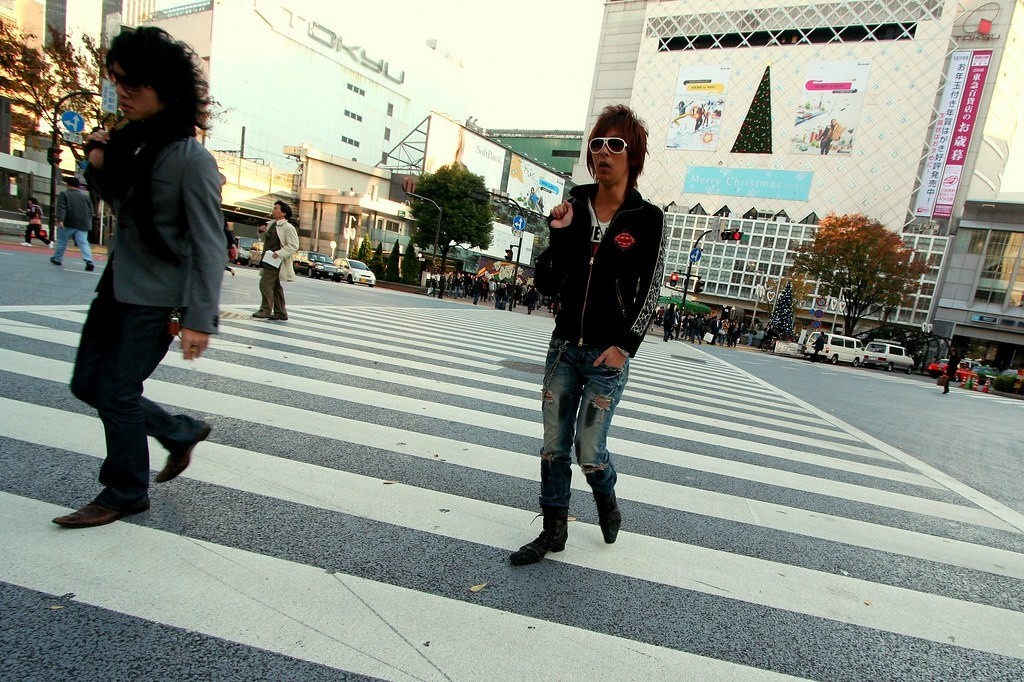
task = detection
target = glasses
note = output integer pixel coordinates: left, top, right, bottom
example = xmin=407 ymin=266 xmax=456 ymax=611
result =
xmin=589 ymin=137 xmax=628 ymax=155
xmin=107 ymin=68 xmax=153 ymax=92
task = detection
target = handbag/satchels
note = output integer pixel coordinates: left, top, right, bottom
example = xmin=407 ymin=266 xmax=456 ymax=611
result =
xmin=753 ymin=331 xmax=757 ymax=335
xmin=718 ymin=329 xmax=726 ymax=336
xmin=533 ymin=197 xmax=576 ymax=298
xmin=937 ymin=375 xmax=949 ymax=386
xmin=703 ymin=332 xmax=714 ymax=343
xmin=229 ymin=246 xmax=236 ymax=260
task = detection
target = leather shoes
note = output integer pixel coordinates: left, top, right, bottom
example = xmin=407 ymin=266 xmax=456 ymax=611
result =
xmin=154 ymin=421 xmax=211 ymax=484
xmin=51 ymin=496 xmax=152 ymax=529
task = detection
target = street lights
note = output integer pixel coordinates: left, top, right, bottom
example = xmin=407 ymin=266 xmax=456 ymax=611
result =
xmin=345 ymin=216 xmax=357 ymax=259
xmin=405 ymin=191 xmax=442 ymax=280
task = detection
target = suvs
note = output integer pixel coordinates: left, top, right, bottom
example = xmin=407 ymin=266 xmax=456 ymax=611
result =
xmin=293 ymin=250 xmax=345 ymax=282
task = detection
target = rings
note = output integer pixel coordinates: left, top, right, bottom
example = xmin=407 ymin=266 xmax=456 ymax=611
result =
xmin=190 ymin=346 xmax=199 ymax=352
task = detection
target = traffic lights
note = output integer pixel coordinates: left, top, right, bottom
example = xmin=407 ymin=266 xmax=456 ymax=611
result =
xmin=670 ymin=273 xmax=678 ymax=287
xmin=504 ymin=249 xmax=513 ymax=262
xmin=721 ymin=231 xmax=744 ymax=240
xmin=468 ymin=189 xmax=490 ymax=202
xmin=47 ymin=147 xmax=64 ymax=165
xmin=694 ymin=280 xmax=704 ymax=293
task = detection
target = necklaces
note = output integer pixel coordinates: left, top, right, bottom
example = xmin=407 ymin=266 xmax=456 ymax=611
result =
xmin=592 ymin=198 xmax=604 ymax=240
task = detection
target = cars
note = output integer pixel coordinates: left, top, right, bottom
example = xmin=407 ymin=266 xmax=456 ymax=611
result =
xmin=333 ymin=257 xmax=377 ymax=287
xmin=927 ymin=357 xmax=1024 ymax=395
xmin=233 ymin=235 xmax=261 ymax=266
xmin=247 ymin=242 xmax=263 ymax=268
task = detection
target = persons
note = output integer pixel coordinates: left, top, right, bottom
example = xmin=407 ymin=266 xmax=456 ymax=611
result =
xmin=942 ymin=349 xmax=961 ymax=394
xmin=252 ymin=200 xmax=299 ymax=321
xmin=765 ymin=324 xmax=774 ymax=350
xmin=225 ymin=217 xmax=238 ymax=276
xmin=812 ymin=332 xmax=825 ymax=364
xmin=49 ymin=177 xmax=94 ymax=271
xmin=425 ymin=267 xmax=749 ymax=349
xmin=816 ymin=119 xmax=837 ymax=155
xmin=508 ymin=105 xmax=670 ymax=567
xmin=20 ymin=198 xmax=54 ymax=250
xmin=51 ymin=26 xmax=230 ymax=527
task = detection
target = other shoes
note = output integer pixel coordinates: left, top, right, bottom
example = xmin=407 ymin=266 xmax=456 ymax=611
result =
xmin=85 ymin=262 xmax=95 ymax=271
xmin=508 ymin=513 xmax=569 ymax=565
xmin=252 ymin=310 xmax=271 ymax=317
xmin=942 ymin=389 xmax=949 ymax=394
xmin=50 ymin=257 xmax=62 ymax=265
xmin=269 ymin=314 xmax=288 ymax=320
xmin=20 ymin=242 xmax=32 ymax=247
xmin=231 ymin=269 xmax=235 ymax=276
xmin=592 ymin=491 xmax=623 ymax=545
xmin=48 ymin=241 xmax=54 ymax=249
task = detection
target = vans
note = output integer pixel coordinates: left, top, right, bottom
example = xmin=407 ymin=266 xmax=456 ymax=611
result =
xmin=861 ymin=338 xmax=914 ymax=374
xmin=801 ymin=331 xmax=865 ymax=367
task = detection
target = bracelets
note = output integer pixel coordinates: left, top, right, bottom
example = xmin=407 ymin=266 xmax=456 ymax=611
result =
xmin=616 ymin=347 xmax=630 ymax=358
